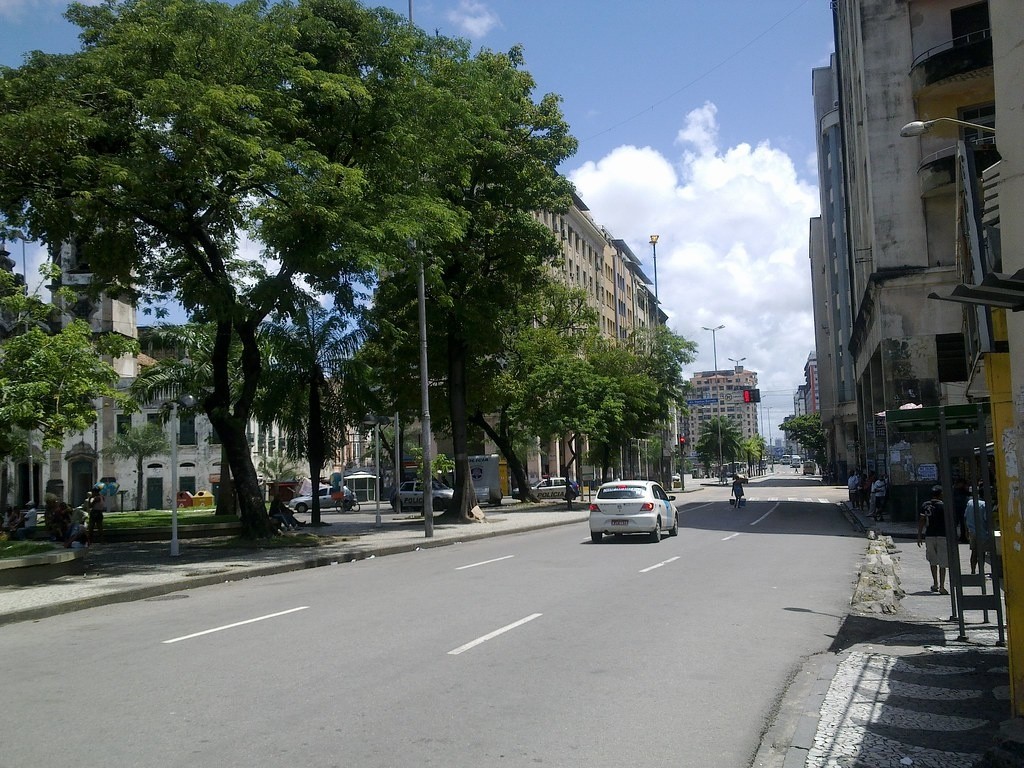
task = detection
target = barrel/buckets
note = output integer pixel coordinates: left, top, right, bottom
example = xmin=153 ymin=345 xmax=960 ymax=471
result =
xmin=739 ymin=498 xmax=746 ymax=508
xmin=729 ymin=499 xmax=735 ymax=505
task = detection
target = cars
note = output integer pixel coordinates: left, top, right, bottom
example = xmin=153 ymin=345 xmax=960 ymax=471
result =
xmin=288 ymin=485 xmax=344 ymax=513
xmin=512 ymin=474 xmax=581 ymax=502
xmin=589 ymin=479 xmax=680 ymax=543
xmin=580 ymin=480 xmax=602 ymax=490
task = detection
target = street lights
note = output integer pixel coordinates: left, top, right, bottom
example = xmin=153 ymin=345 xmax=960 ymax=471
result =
xmin=169 ymin=395 xmax=196 ymax=558
xmin=649 ymin=234 xmax=661 ymax=344
xmin=728 ymin=357 xmax=747 ymax=436
xmin=702 ymin=325 xmax=727 ymax=483
xmin=763 ymin=406 xmax=773 ymax=460
xmin=759 ymin=395 xmax=766 ymax=439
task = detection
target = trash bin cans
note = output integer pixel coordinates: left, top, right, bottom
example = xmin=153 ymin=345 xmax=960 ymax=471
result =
xmin=674 ymin=477 xmax=681 ymax=487
xmin=177 ymin=490 xmax=193 ymax=507
xmin=192 ymin=490 xmax=215 ymax=507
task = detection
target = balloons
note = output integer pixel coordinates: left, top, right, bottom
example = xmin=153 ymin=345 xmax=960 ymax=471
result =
xmin=93 ymin=481 xmax=120 ymax=497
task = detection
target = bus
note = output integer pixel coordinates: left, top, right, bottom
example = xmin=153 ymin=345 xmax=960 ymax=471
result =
xmin=693 ymin=461 xmax=749 ymax=478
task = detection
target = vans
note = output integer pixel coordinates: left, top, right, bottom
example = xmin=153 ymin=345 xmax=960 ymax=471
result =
xmin=803 ymin=461 xmax=816 ymax=475
xmin=767 ymin=454 xmax=801 ymax=468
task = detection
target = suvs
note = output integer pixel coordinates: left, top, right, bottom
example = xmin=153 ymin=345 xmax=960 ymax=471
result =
xmin=390 ymin=479 xmax=454 ymax=514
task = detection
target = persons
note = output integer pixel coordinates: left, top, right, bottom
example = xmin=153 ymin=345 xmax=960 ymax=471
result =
xmin=712 ymin=471 xmax=714 ymax=478
xmin=50 ymin=501 xmax=90 ymax=548
xmin=952 ymin=471 xmax=996 ymax=574
xmin=731 ymin=475 xmax=745 ymax=508
xmin=269 ymin=493 xmax=306 ymax=531
xmin=917 ymin=485 xmax=949 ymax=594
xmin=848 ymin=468 xmax=887 ymax=516
xmin=88 ymin=489 xmax=105 ymax=542
xmin=0 ymin=500 xmax=37 ymax=541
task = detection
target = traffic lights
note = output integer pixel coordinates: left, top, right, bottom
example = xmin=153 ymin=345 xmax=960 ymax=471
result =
xmin=680 ymin=437 xmax=685 ymax=449
xmin=743 ymin=389 xmax=761 ymax=403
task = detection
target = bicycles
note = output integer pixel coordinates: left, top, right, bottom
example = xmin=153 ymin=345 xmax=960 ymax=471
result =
xmin=335 ymin=493 xmax=361 ymax=514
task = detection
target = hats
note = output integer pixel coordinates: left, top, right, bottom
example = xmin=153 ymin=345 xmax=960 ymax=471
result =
xmin=931 ymin=485 xmax=942 ymax=496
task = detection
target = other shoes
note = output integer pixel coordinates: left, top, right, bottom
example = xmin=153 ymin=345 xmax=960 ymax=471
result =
xmin=939 ymin=588 xmax=949 ymax=594
xmin=931 ymin=585 xmax=939 ymax=591
